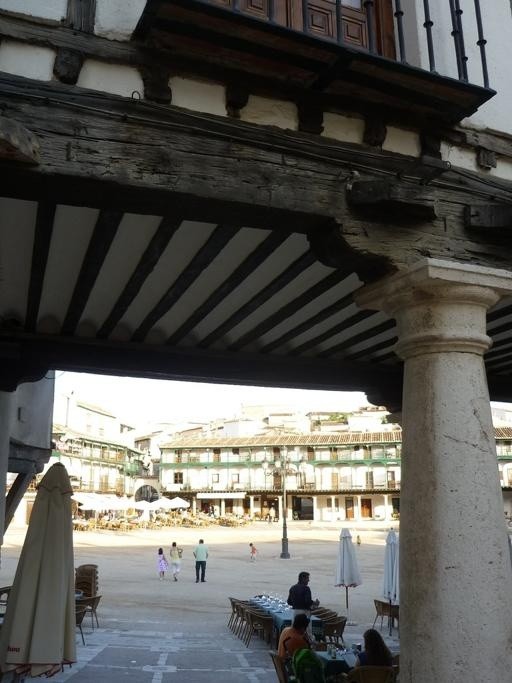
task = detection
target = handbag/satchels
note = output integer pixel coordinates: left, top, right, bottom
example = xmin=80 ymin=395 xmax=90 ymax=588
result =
xmin=178 ymin=552 xmax=183 ymax=558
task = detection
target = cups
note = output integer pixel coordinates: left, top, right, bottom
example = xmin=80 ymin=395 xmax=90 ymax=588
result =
xmin=356 ymin=642 xmax=361 ymax=652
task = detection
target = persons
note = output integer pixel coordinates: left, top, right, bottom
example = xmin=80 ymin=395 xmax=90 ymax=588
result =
xmin=352 ymin=628 xmax=394 ymax=666
xmin=286 ymin=571 xmax=320 ymax=640
xmin=248 ymin=542 xmax=258 ymax=564
xmin=170 ymin=541 xmax=184 ymax=582
xmin=157 ymin=549 xmax=168 ymax=581
xmin=276 ymin=614 xmax=313 ymax=673
xmin=192 ymin=538 xmax=211 ymax=583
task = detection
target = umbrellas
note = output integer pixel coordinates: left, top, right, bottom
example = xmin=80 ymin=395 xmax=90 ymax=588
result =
xmin=383 ymin=526 xmax=398 ymax=639
xmin=335 ymin=527 xmax=362 ymax=625
xmin=0 ymin=462 xmax=77 ymax=678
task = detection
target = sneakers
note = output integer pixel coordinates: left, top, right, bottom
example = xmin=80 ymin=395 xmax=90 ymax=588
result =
xmin=195 ymin=580 xmax=199 ymax=583
xmin=201 ymin=580 xmax=207 ymax=583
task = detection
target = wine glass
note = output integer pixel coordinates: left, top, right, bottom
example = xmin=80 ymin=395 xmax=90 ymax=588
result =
xmin=260 ymin=590 xmax=294 ymax=614
xmin=334 ymin=646 xmax=347 ymax=656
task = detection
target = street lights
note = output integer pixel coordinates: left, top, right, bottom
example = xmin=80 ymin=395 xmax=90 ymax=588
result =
xmin=262 ymin=445 xmax=308 ymax=558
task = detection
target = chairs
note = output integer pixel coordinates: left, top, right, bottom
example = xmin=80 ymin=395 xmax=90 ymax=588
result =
xmin=226 ymin=593 xmax=399 ymax=682
xmin=1 ymin=563 xmax=102 ymax=682
xmin=72 ymin=508 xmax=275 ymax=533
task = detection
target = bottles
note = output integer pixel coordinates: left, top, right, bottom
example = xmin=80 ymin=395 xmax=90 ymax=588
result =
xmin=327 ymin=639 xmax=336 ymax=659
xmin=386 ymin=591 xmax=396 ymax=603
